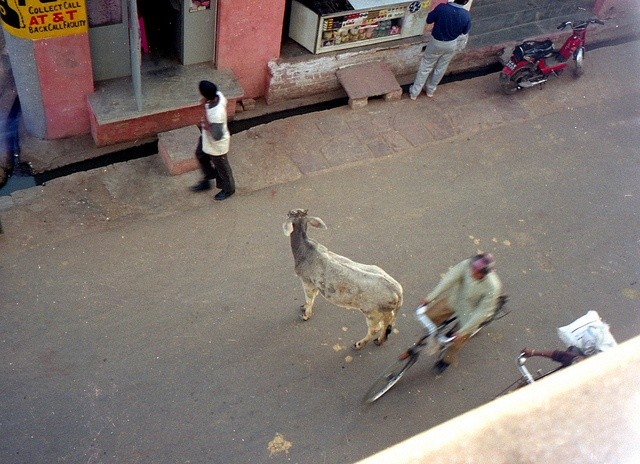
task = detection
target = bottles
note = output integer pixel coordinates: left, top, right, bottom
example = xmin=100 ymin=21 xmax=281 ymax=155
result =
xmin=375 ymin=22 xmax=380 ymax=37
xmin=380 ymin=21 xmax=386 ymax=37
xmin=386 ymin=20 xmax=393 ymax=35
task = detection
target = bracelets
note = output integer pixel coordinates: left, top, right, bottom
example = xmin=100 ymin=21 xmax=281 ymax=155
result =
xmin=530 ymin=348 xmax=536 ymax=357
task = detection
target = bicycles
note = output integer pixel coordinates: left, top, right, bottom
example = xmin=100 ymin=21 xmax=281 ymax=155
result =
xmin=363 ymin=295 xmax=510 ymax=404
xmin=495 ymin=351 xmax=553 ymax=397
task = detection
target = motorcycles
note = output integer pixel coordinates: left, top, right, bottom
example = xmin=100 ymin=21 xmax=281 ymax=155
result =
xmin=499 ymin=18 xmax=605 ymax=95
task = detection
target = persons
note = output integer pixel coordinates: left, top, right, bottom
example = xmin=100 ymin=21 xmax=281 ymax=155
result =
xmin=409 ymin=0 xmax=472 ymax=103
xmin=190 ymin=81 xmax=236 ymax=200
xmin=518 ymin=345 xmax=590 ymax=381
xmin=417 ymin=252 xmax=502 ymax=375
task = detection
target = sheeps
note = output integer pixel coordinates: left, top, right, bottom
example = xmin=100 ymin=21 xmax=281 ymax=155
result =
xmin=282 ymin=208 xmax=404 ymax=351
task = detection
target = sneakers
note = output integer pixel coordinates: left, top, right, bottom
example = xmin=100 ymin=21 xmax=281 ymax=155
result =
xmin=215 ymin=189 xmax=235 ymax=200
xmin=191 ymin=181 xmax=210 ymax=190
xmin=432 ymin=359 xmax=449 ymax=374
xmin=423 ymin=85 xmax=433 ymax=97
xmin=409 ymin=85 xmax=416 ymax=100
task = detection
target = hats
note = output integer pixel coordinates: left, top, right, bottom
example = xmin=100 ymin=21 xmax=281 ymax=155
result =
xmin=472 ymin=252 xmax=495 ymax=273
